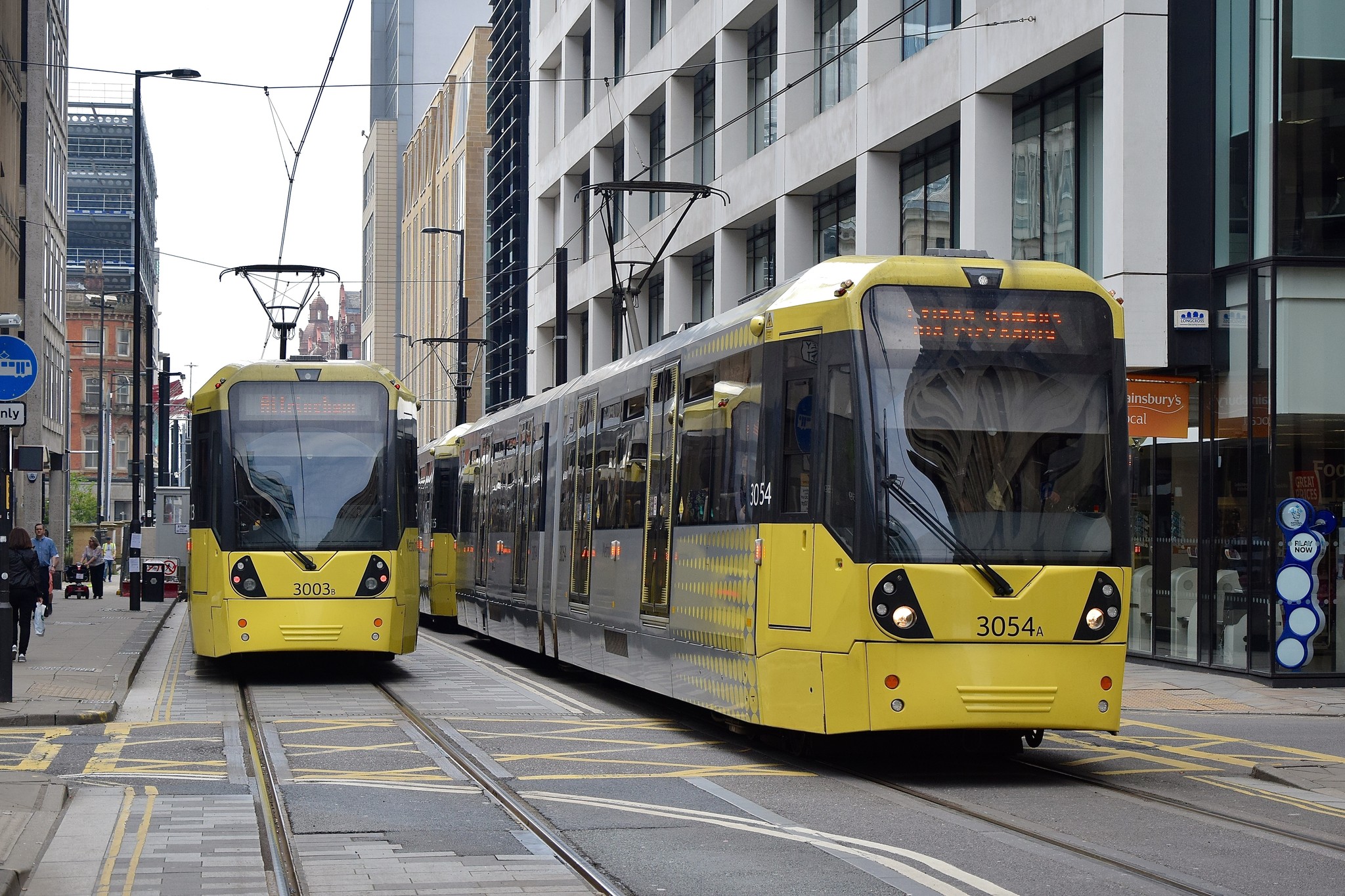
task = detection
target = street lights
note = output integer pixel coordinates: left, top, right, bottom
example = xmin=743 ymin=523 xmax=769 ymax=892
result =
xmin=97 ymin=288 xmax=144 ymax=524
xmin=419 ymin=226 xmax=465 ymax=427
xmin=130 ymin=67 xmax=202 ymax=611
xmin=183 ymin=362 xmax=199 ymax=442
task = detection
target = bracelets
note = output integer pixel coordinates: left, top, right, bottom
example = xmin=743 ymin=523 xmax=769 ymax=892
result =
xmin=113 ymin=555 xmax=116 ymax=556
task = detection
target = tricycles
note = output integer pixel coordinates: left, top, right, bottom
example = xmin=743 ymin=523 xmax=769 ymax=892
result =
xmin=64 ymin=563 xmax=90 ymax=599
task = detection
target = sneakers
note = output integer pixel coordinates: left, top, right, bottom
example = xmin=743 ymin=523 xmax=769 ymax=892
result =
xmin=18 ymin=653 xmax=27 ymax=662
xmin=48 ymin=604 xmax=52 ymax=615
xmin=103 ymin=577 xmax=106 ymax=582
xmin=12 ymin=645 xmax=18 ymax=661
xmin=43 ymin=609 xmax=48 ymax=618
xmin=33 ymin=610 xmax=36 ymax=619
xmin=108 ymin=578 xmax=112 ymax=582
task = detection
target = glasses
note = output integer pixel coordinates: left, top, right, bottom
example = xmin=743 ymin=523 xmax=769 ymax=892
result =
xmin=35 ymin=528 xmax=44 ymax=530
xmin=89 ymin=540 xmax=94 ymax=542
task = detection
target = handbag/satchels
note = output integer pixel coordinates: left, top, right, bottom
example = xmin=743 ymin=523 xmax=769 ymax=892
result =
xmin=33 ymin=602 xmax=47 ymax=637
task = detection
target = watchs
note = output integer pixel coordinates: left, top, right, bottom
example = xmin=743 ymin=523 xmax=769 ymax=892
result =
xmin=51 ymin=565 xmax=56 ymax=569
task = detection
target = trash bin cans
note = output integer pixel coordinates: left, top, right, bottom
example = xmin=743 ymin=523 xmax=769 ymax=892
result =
xmin=142 ymin=560 xmax=165 ymax=602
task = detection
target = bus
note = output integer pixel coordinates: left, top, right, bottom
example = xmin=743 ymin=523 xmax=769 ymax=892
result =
xmin=182 ymin=354 xmax=426 ymax=671
xmin=420 ymin=241 xmax=1134 ymax=753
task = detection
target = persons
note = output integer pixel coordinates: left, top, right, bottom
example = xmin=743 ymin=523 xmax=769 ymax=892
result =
xmin=30 ymin=523 xmax=59 ymax=620
xmin=41 ymin=529 xmax=60 ymax=615
xmin=8 ymin=526 xmax=45 ymax=661
xmin=101 ymin=536 xmax=117 ymax=582
xmin=953 ymin=410 xmax=1063 ymax=514
xmin=80 ymin=536 xmax=105 ymax=600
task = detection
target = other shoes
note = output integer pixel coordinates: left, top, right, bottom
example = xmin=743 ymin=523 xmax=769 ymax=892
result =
xmin=93 ymin=594 xmax=97 ymax=599
xmin=99 ymin=596 xmax=102 ymax=599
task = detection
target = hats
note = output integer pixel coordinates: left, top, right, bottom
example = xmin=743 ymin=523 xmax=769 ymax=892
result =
xmin=106 ymin=536 xmax=111 ymax=539
